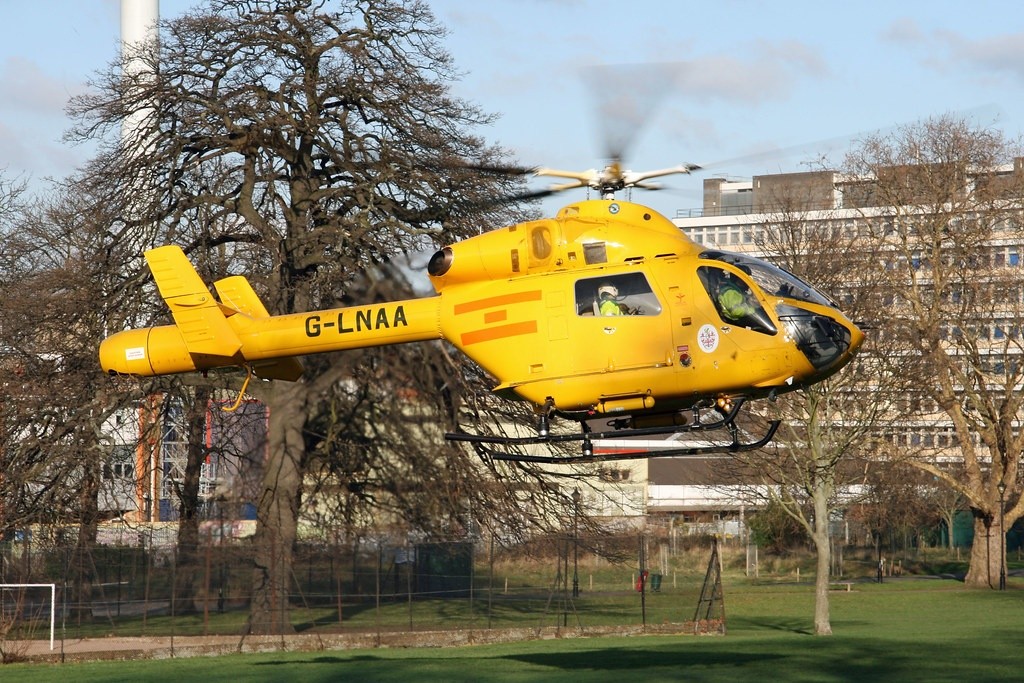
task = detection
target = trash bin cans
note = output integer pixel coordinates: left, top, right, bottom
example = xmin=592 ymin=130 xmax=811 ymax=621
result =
xmin=650 ymin=573 xmax=662 ymax=592
xmin=637 ymin=571 xmax=649 ymax=592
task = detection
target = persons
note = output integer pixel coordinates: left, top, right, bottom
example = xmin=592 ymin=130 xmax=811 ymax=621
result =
xmin=717 ymin=263 xmax=756 ymax=321
xmin=597 ymin=279 xmax=631 ymax=316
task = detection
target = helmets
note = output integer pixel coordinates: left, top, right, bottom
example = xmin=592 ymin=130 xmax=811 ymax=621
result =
xmin=598 ymin=281 xmax=618 ymax=300
xmin=730 ymin=265 xmax=751 ymax=281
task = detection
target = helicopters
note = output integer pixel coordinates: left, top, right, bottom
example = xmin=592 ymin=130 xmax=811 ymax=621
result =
xmin=96 ymin=102 xmax=985 ymax=465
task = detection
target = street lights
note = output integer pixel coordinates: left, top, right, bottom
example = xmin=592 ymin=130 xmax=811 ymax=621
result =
xmin=216 ymin=494 xmax=229 ymax=612
xmin=873 ymin=483 xmax=884 ymax=575
xmin=995 ymin=480 xmax=1007 ymax=590
xmin=571 ymin=489 xmax=582 ymax=595
